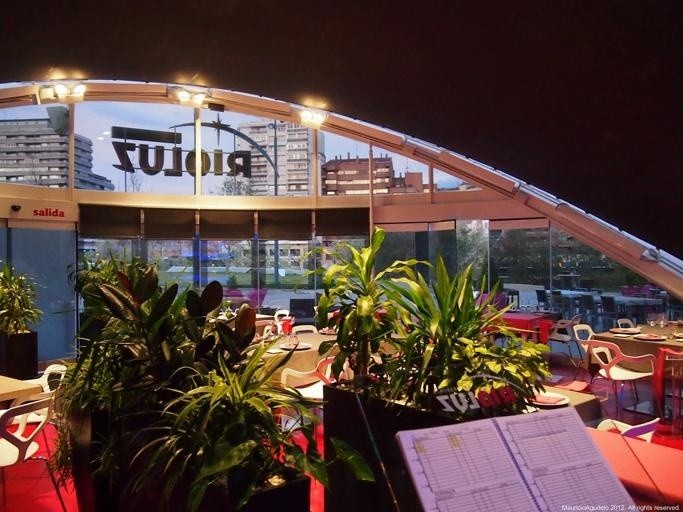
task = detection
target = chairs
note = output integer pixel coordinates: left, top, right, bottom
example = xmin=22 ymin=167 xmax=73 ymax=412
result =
xmin=0 ymin=276 xmax=682 ymax=511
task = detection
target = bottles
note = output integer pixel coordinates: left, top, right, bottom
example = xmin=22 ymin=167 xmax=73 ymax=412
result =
xmin=225 ymin=310 xmax=232 ymax=319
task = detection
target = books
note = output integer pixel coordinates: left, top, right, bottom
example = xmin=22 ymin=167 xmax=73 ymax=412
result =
xmin=395 ymin=406 xmax=639 ymax=512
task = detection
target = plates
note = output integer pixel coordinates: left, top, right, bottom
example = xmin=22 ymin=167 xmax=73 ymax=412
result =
xmin=319 ymin=329 xmax=338 ymax=335
xmin=523 ymin=392 xmax=569 ymax=406
xmin=609 ymin=320 xmax=682 ymax=340
xmin=280 ymin=343 xmax=311 ymax=349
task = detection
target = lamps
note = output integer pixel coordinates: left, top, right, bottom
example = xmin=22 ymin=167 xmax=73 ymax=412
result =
xmin=52 ymin=79 xmax=87 ymax=100
xmin=165 ymin=82 xmax=213 ymax=107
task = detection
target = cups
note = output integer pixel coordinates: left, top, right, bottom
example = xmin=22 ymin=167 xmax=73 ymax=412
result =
xmin=645 ymin=313 xmax=667 ymax=328
xmin=286 ymin=335 xmax=298 ymax=347
